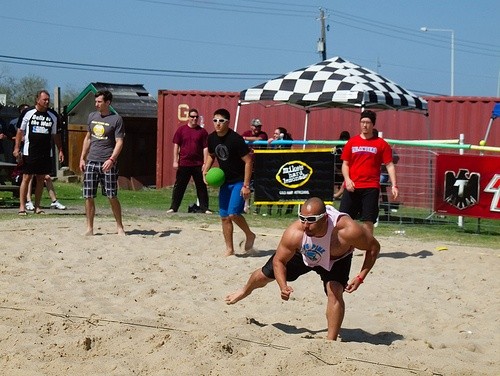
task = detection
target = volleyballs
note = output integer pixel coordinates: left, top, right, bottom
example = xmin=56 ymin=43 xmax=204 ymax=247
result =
xmin=205 ymin=168 xmax=226 ymax=187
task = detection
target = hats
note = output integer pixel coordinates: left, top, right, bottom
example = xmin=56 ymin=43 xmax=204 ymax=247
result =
xmin=251 ymin=119 xmax=263 ymax=126
xmin=360 ymin=110 xmax=376 ymax=126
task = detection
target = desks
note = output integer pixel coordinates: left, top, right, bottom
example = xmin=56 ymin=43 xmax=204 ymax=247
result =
xmin=333 ymin=180 xmax=392 ymax=214
xmin=0 ymin=161 xmax=19 ymax=197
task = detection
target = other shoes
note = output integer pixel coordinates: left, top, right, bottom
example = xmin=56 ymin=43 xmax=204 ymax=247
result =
xmin=36 ymin=211 xmax=45 ymax=214
xmin=253 ymin=208 xmax=260 ymax=213
xmin=267 ymin=209 xmax=271 ymax=215
xmin=19 ymin=211 xmax=27 ymax=215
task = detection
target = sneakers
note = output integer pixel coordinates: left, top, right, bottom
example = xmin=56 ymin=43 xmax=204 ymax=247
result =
xmin=25 ymin=201 xmax=35 ymax=211
xmin=50 ymin=201 xmax=66 ymax=209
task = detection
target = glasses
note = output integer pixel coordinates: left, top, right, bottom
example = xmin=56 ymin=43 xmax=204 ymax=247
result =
xmin=190 ymin=116 xmax=199 ymax=118
xmin=362 ymin=121 xmax=371 ymax=124
xmin=213 ymin=119 xmax=228 ymax=123
xmin=299 ymin=214 xmax=324 ymax=223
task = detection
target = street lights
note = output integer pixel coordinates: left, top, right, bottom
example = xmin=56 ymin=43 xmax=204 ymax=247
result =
xmin=419 ymin=26 xmax=455 ymax=97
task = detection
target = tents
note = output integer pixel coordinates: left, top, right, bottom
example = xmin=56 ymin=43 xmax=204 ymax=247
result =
xmin=234 ymin=56 xmax=432 ymax=224
xmin=480 ymin=103 xmax=500 ymax=156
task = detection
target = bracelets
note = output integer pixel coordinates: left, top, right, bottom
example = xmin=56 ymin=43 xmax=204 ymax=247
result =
xmin=358 ymin=275 xmax=363 ymax=283
xmin=203 ymin=163 xmax=206 ymax=164
xmin=109 ymin=157 xmax=115 ymax=162
xmin=391 ymin=185 xmax=398 ymax=188
xmin=243 ymin=136 xmax=246 ymax=140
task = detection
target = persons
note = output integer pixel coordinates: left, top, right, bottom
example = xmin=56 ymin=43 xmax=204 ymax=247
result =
xmin=225 ymin=197 xmax=380 ymax=340
xmin=203 ymin=109 xmax=255 ymax=256
xmin=241 ymin=118 xmax=267 ymax=213
xmin=79 ymin=89 xmax=126 ymax=237
xmin=333 ymin=131 xmax=350 ymax=182
xmin=166 ymin=109 xmax=213 ymax=214
xmin=9 ymin=147 xmax=67 ymax=211
xmin=372 ymin=129 xmax=400 ymax=221
xmin=0 ymin=104 xmax=29 ymax=151
xmin=262 ymin=127 xmax=292 ymax=217
xmin=13 ymin=88 xmax=64 ymax=217
xmin=339 ymin=111 xmax=399 ymax=256
xmin=243 ymin=185 xmax=250 ymax=189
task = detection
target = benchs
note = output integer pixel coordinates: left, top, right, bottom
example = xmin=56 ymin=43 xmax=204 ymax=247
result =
xmin=0 ymin=183 xmax=20 ymax=191
xmin=379 ymin=200 xmax=400 ymax=210
